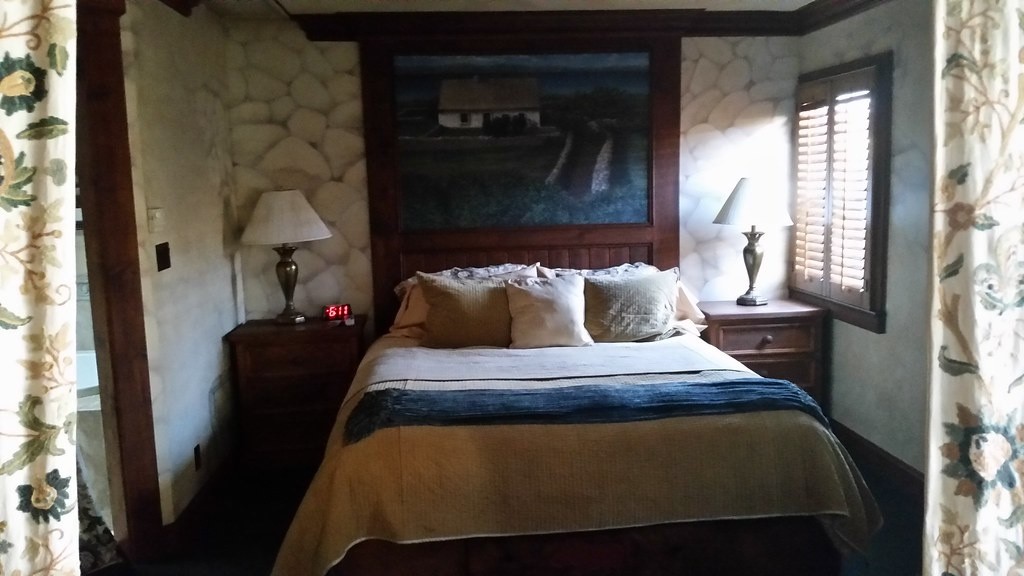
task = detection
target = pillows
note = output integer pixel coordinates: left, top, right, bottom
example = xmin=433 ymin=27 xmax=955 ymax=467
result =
xmin=387 ymin=260 xmax=680 ymax=358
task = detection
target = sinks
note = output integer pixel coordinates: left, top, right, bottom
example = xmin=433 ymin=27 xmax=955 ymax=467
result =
xmin=73 ymin=350 xmax=99 ymax=397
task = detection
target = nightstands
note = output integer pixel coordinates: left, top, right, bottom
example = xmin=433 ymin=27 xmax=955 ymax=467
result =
xmin=226 ymin=309 xmax=371 ymax=461
xmin=698 ymin=297 xmax=826 ymax=402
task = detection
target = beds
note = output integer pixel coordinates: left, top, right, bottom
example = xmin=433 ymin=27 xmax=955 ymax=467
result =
xmin=269 ymin=244 xmax=890 ymax=576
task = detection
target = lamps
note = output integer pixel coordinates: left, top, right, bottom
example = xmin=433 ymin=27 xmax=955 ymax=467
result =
xmin=240 ymin=189 xmax=333 ymax=321
xmin=714 ymin=179 xmax=795 ymax=306
